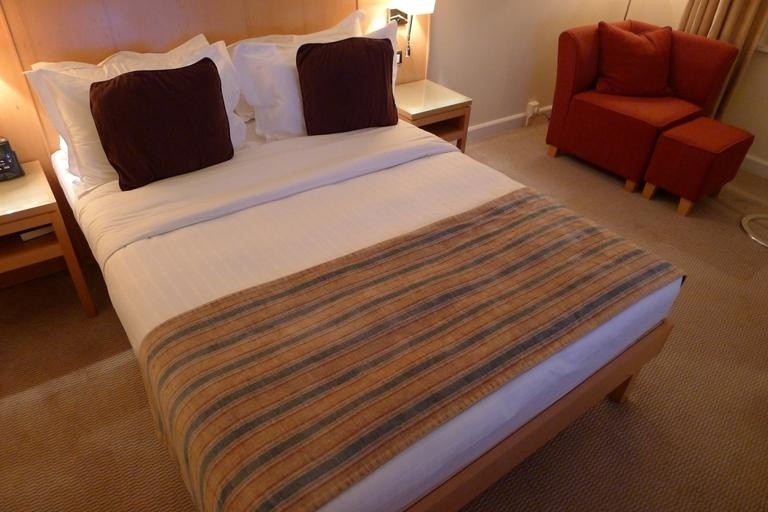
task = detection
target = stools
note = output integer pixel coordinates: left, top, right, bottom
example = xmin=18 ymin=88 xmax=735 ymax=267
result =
xmin=639 ymin=117 xmax=756 ymax=214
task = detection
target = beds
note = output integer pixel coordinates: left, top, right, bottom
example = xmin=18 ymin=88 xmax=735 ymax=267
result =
xmin=51 ymin=122 xmax=691 ymax=512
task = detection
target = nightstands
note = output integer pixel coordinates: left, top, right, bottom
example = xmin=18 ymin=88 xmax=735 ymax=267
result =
xmin=392 ymin=77 xmax=474 ymax=152
xmin=2 ymin=160 xmax=99 ymax=318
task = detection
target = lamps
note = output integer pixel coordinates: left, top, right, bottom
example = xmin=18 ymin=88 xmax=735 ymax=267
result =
xmin=388 ymin=1 xmax=436 ymax=28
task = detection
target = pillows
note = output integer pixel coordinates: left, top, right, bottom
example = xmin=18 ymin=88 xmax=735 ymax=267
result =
xmin=232 ymin=20 xmax=398 ymax=141
xmin=296 ymin=38 xmax=398 ymax=135
xmin=89 ymin=57 xmax=235 ymax=191
xmin=20 ymin=40 xmax=248 ymax=198
xmin=30 ymin=33 xmax=210 ymax=74
xmin=595 ymin=20 xmax=678 ymax=97
xmin=227 ymin=10 xmax=367 ymax=121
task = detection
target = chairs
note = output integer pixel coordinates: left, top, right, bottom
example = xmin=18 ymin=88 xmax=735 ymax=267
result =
xmin=547 ymin=18 xmax=742 ymax=194
